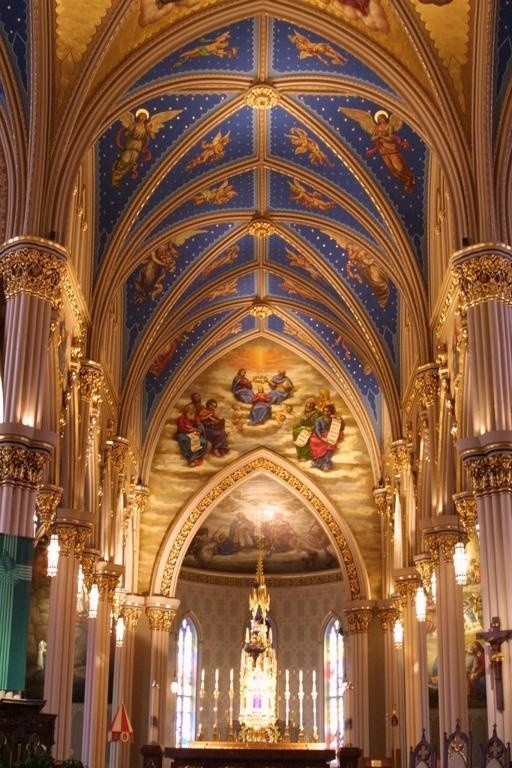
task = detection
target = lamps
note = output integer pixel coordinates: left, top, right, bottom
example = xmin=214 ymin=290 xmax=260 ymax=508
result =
xmin=241 ymin=512 xmax=273 ymax=658
xmin=46 ymin=507 xmax=127 ymax=647
xmin=393 ymin=513 xmax=467 ymax=648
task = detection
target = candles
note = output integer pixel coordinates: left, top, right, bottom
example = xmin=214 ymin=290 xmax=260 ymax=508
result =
xmin=199 ymin=665 xmax=318 ymax=725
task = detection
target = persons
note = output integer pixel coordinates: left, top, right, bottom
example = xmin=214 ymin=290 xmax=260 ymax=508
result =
xmin=200 ymin=398 xmax=230 ymax=458
xmin=230 ymin=511 xmax=256 ymax=549
xmin=263 ymin=368 xmax=294 ymax=407
xmin=293 ymin=397 xmax=320 ymax=462
xmin=250 ymin=385 xmax=273 ymax=426
xmin=308 ymin=402 xmax=345 ymax=471
xmin=176 ymin=403 xmax=209 ymax=468
xmin=189 ymin=392 xmax=204 ymax=413
xmin=232 ymin=369 xmax=255 ymax=402
xmin=263 ymin=512 xmax=297 ymax=555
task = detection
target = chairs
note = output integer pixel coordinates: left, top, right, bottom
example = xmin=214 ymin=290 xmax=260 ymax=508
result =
xmin=409 ymin=718 xmax=512 ymax=768
